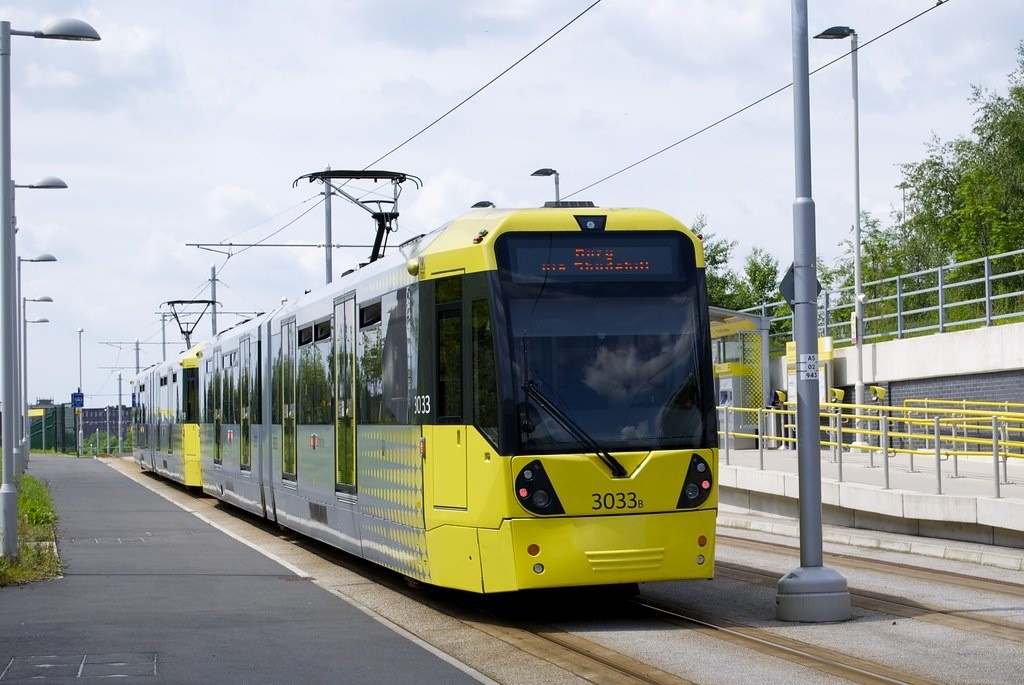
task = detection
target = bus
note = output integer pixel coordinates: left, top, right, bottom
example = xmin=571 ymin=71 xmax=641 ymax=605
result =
xmin=127 ymin=206 xmax=721 ymax=603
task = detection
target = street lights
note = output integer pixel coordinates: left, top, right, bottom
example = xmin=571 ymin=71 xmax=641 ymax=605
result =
xmin=533 ymin=165 xmax=562 ymax=199
xmin=0 ymin=15 xmax=102 ymax=561
xmin=76 ymin=327 xmax=85 ymax=456
xmin=811 ymin=25 xmax=877 ymax=451
